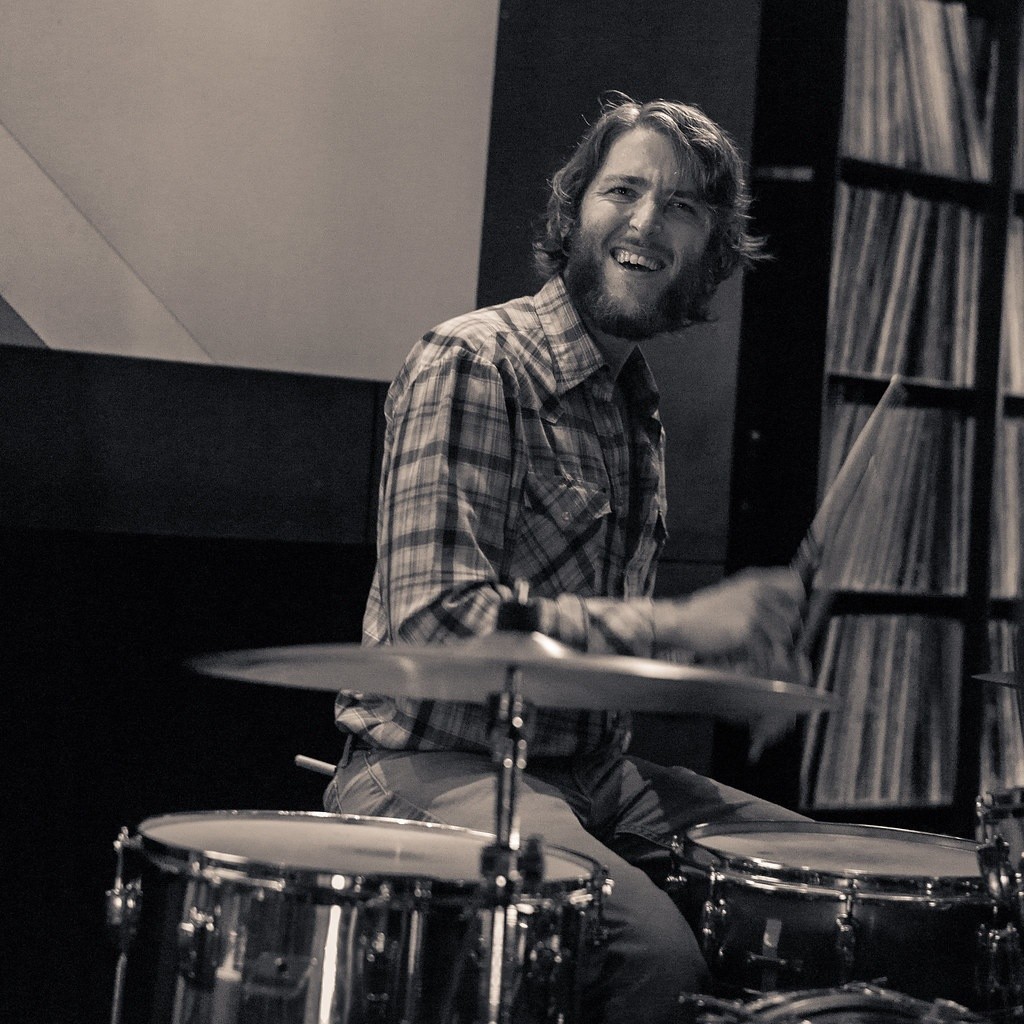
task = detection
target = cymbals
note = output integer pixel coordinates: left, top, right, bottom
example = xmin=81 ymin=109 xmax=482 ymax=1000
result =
xmin=186 ymin=633 xmax=842 ymax=718
xmin=972 ymin=669 xmax=1023 ymax=690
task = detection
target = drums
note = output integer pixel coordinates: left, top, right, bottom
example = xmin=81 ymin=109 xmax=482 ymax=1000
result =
xmin=96 ymin=808 xmax=611 ymax=1024
xmin=664 ymin=816 xmax=1009 ymax=993
xmin=689 ymin=978 xmax=973 ymax=1023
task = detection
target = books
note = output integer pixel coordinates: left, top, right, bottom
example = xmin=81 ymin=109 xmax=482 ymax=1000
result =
xmin=825 ymin=183 xmax=1022 ymax=394
xmin=822 ymin=403 xmax=1023 ymax=601
xmin=800 ymin=614 xmax=1024 ymax=807
xmin=840 ymin=0 xmax=990 ymax=184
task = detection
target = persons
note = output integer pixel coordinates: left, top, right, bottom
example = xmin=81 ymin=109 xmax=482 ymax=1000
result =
xmin=318 ymin=102 xmax=816 ymax=1024
